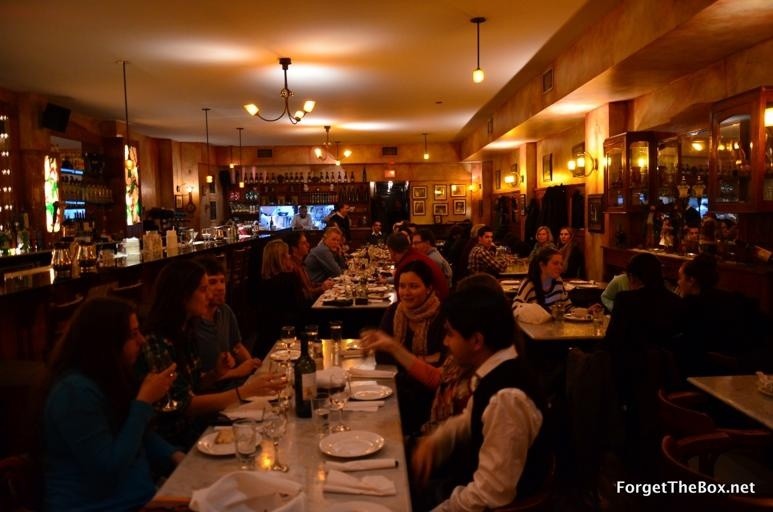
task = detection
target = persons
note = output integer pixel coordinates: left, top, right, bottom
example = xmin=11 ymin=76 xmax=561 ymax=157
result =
xmin=360 ymin=273 xmax=525 ymax=451
xmin=144 ymin=261 xmax=288 ymax=412
xmin=408 ymin=288 xmax=550 ymax=512
xmin=35 ymin=295 xmax=187 ymax=511
xmin=186 ymin=257 xmax=263 ymax=388
xmin=262 ymin=200 xmax=736 ymax=396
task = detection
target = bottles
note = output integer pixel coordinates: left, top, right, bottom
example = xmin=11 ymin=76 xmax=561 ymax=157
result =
xmin=163 ymin=221 xmax=239 ymax=258
xmin=615 ymin=164 xmax=772 ymax=203
xmin=59 ymin=150 xmax=112 ymax=237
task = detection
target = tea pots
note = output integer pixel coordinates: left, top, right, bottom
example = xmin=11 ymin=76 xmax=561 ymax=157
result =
xmin=50 ymin=240 xmax=98 ymax=274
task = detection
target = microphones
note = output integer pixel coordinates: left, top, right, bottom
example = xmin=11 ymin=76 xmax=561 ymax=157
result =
xmin=53 ymin=201 xmax=60 ymax=224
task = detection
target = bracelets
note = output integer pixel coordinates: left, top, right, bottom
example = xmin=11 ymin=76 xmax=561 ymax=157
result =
xmin=235 ymin=387 xmax=245 ymax=404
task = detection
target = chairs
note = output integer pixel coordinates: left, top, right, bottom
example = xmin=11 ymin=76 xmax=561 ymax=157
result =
xmin=0 ymin=450 xmax=40 ymax=512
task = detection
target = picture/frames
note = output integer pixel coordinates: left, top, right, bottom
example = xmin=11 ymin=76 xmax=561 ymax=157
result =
xmin=412 ymin=184 xmax=466 ymax=216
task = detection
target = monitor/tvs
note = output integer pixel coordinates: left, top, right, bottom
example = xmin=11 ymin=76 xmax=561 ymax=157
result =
xmin=40 ymin=102 xmax=72 ymax=133
xmin=297 ymin=203 xmax=336 ymax=232
xmin=257 ymin=204 xmax=297 ymax=232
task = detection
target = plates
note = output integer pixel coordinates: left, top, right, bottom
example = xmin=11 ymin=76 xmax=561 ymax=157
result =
xmin=230 ymin=168 xmax=365 ymax=217
xmin=564 ymin=302 xmax=596 ymax=324
xmin=320 ymin=240 xmax=394 ymax=307
xmin=502 ymin=278 xmax=599 ymax=291
xmin=198 ymin=320 xmax=394 ymax=473
